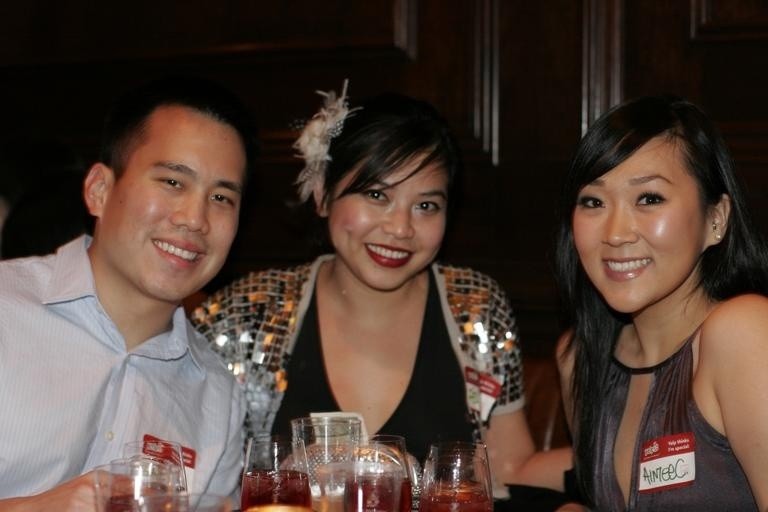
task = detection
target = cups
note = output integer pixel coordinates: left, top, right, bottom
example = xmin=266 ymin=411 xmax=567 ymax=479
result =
xmin=419 ymin=440 xmax=494 ymax=509
xmin=239 ymin=416 xmax=412 ymax=511
xmin=92 ymin=439 xmax=189 ymax=511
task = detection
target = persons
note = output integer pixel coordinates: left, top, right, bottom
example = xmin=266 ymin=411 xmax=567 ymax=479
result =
xmin=187 ymin=77 xmax=574 ymax=501
xmin=0 ymin=97 xmax=250 ymax=512
xmin=553 ymin=90 xmax=767 ymax=511
